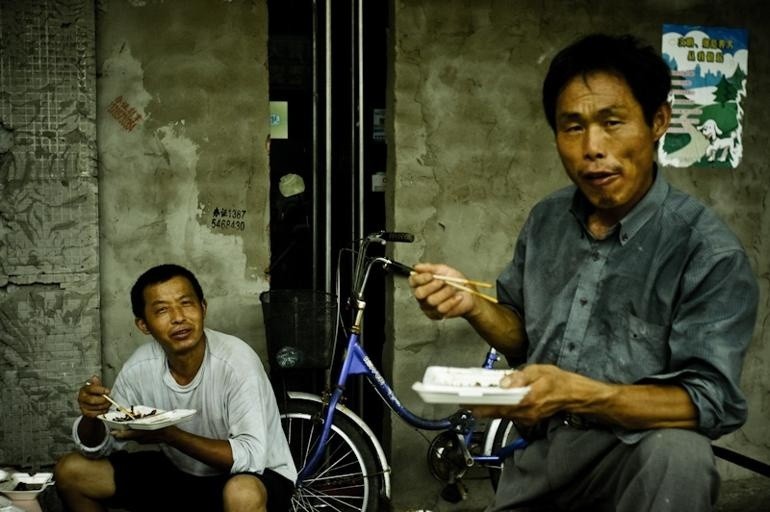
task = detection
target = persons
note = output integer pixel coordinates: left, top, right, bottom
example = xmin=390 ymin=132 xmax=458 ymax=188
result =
xmin=55 ymin=261 xmax=298 ymax=512
xmin=407 ymin=31 xmax=761 ymax=512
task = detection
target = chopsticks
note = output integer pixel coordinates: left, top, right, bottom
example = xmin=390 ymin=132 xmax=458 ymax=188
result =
xmin=410 ymin=271 xmax=499 ymax=305
xmin=85 ymin=382 xmax=136 ymax=421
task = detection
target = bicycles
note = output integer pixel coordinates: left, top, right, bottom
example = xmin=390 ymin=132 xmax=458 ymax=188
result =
xmin=276 ymin=229 xmax=530 ymax=511
